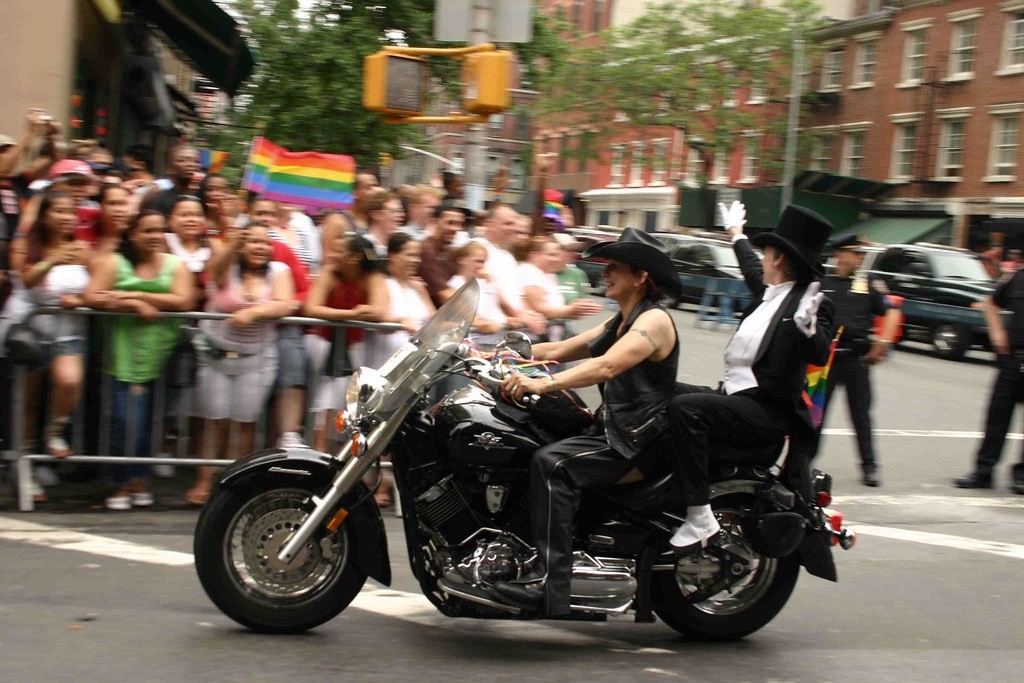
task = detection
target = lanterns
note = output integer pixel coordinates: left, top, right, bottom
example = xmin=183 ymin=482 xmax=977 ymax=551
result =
xmin=95 ymin=127 xmax=106 ymax=135
xmin=97 ymin=108 xmax=106 ymax=116
xmin=72 ymin=96 xmax=82 ymax=106
xmin=69 ymin=115 xmax=80 ymax=129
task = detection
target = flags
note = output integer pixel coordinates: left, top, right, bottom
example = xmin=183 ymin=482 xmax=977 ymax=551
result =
xmin=242 ymin=134 xmax=355 ymax=209
xmin=199 ymin=147 xmax=232 ymax=173
xmin=785 ymin=339 xmax=838 ymax=440
xmin=541 ymin=188 xmax=563 ymax=221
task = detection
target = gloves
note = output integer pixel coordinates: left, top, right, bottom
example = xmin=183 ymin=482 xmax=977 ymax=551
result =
xmin=720 ymin=199 xmax=747 ymax=227
xmin=793 ymin=282 xmax=824 ymax=324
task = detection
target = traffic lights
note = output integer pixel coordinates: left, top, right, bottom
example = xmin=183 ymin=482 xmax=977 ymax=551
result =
xmin=462 ymin=49 xmax=515 ymax=112
xmin=362 ymin=50 xmax=429 ymax=114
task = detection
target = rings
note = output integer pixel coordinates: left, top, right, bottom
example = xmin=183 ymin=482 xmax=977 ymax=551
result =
xmin=513 ymin=385 xmax=517 ymax=390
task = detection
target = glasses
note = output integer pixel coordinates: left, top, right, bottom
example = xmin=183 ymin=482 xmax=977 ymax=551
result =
xmin=55 ymin=175 xmax=92 ymax=187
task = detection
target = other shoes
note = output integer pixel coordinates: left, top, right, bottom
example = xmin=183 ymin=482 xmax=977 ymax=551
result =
xmin=953 ymin=471 xmax=992 ymax=488
xmin=7 ymin=432 xmax=218 ymax=510
xmin=656 ymin=524 xmax=721 ymax=556
xmin=1013 ymin=475 xmax=1024 ymax=494
xmin=861 ymin=462 xmax=878 ymax=486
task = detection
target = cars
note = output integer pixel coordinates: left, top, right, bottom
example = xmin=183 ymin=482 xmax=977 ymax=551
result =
xmin=567 ymin=224 xmax=1014 ymax=359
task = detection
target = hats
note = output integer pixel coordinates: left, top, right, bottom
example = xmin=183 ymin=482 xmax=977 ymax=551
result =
xmin=580 ymin=226 xmax=685 ymax=298
xmin=753 ymin=202 xmax=832 ymax=280
xmin=551 ymin=233 xmax=582 ymax=251
xmin=824 ymin=231 xmax=862 ymax=251
xmin=42 ymin=160 xmax=91 ymax=178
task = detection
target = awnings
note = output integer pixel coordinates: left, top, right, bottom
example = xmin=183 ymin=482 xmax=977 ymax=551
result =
xmin=118 ymin=0 xmax=256 ymax=99
xmin=825 ymin=215 xmax=950 ymax=245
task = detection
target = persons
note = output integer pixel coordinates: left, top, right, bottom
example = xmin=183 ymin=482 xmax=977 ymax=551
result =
xmin=0 ymin=108 xmax=610 ymax=507
xmin=865 ymin=280 xmax=904 ymax=363
xmin=779 ymin=231 xmax=901 ymax=490
xmin=949 ymin=243 xmax=1024 ymax=494
xmin=666 ymin=203 xmax=833 ymax=550
xmin=453 ymin=228 xmax=681 ymax=618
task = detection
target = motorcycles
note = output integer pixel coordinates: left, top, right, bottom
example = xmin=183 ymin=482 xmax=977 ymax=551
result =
xmin=193 ymin=279 xmax=855 ymax=640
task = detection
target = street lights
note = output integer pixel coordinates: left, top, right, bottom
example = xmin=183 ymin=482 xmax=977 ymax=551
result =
xmin=744 ymin=95 xmax=800 ymax=214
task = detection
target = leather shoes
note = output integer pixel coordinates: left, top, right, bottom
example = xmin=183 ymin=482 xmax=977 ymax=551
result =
xmin=494 ymin=581 xmax=547 ymax=611
